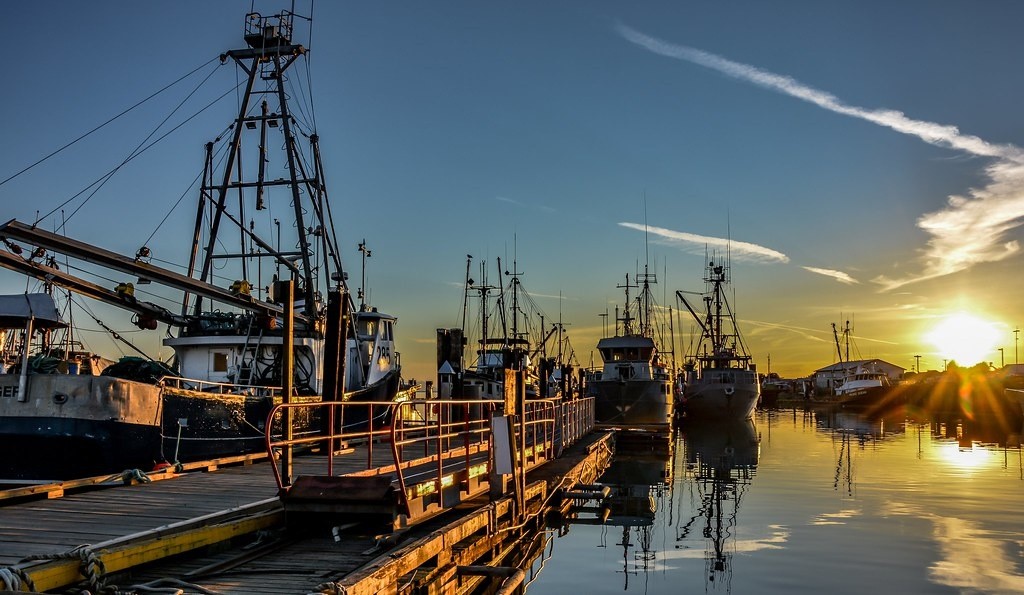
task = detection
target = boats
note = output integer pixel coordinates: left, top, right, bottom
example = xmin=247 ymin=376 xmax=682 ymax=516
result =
xmin=437 ymin=232 xmax=583 ymax=436
xmin=584 ymin=194 xmax=677 ymax=439
xmin=675 ymin=420 xmax=762 ymax=580
xmin=674 ymin=209 xmax=762 ymax=433
xmin=597 ymin=438 xmax=672 ymax=593
xmin=1 ymin=1 xmax=403 ymax=470
